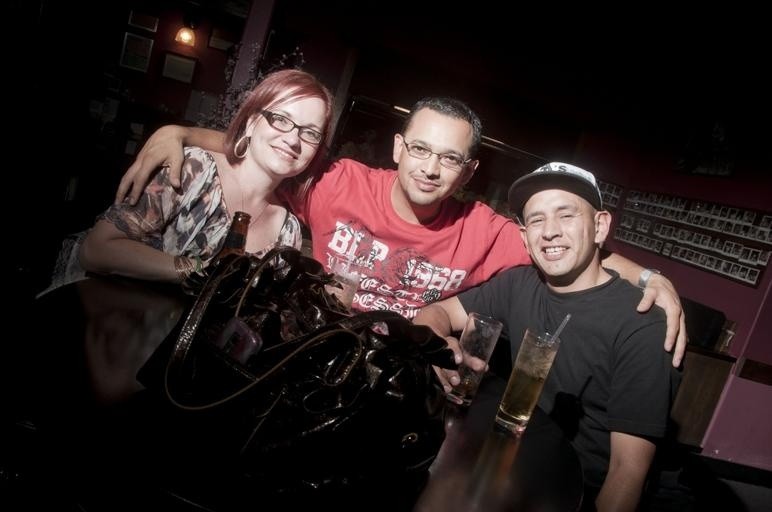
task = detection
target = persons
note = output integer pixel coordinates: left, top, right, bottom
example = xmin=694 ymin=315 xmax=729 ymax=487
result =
xmin=81 ymin=276 xmax=188 ymax=402
xmin=406 ymin=159 xmax=686 ymax=510
xmin=112 ymin=95 xmax=689 ymax=369
xmin=409 ymin=383 xmax=587 ymax=510
xmin=30 ymin=67 xmax=338 ymax=298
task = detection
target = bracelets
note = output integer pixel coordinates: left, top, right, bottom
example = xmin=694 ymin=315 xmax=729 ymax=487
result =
xmin=636 ymin=268 xmax=662 ymax=289
xmin=192 ymin=254 xmax=202 ymax=271
xmin=172 ymin=254 xmax=195 ymax=283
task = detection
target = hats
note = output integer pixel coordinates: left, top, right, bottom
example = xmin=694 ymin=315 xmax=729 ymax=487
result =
xmin=506 ymin=160 xmax=605 ymax=224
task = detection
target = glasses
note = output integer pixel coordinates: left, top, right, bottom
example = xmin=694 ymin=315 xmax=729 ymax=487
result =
xmin=400 ymin=132 xmax=473 ymax=170
xmin=254 ymin=106 xmax=323 ymax=145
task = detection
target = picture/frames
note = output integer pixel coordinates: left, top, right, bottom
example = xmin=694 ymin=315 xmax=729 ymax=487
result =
xmin=119 ymin=10 xmax=241 ymax=84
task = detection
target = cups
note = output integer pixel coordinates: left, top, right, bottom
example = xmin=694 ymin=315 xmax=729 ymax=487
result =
xmin=444 ymin=310 xmax=505 ymax=410
xmin=492 ymin=327 xmax=562 ymax=435
xmin=324 ymin=273 xmax=357 ymax=312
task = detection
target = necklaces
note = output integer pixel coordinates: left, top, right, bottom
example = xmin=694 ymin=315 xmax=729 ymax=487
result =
xmin=236 ymin=165 xmax=275 ymax=229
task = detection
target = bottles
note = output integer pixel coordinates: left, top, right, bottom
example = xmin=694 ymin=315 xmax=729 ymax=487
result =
xmin=207 ymin=209 xmax=251 ymax=281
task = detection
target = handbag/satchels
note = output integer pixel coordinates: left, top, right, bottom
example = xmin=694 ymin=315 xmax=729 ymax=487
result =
xmin=178 ymin=252 xmax=456 ymax=510
